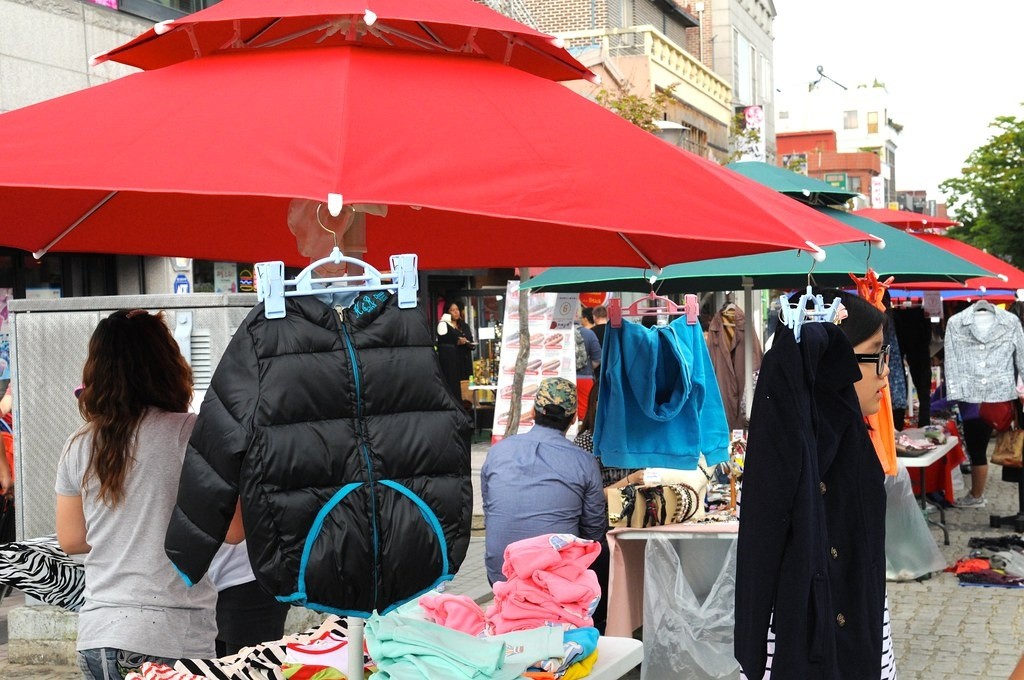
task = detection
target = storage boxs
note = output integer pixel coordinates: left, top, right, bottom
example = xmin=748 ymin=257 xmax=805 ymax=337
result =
xmin=460 ymin=380 xmax=494 ymax=402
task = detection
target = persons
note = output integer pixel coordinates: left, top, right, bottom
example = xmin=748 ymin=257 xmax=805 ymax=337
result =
xmin=929 ymin=330 xmax=995 ymax=508
xmin=0 ymin=380 xmax=14 ymax=521
xmin=443 ymin=301 xmax=477 ymax=381
xmin=480 ymin=377 xmax=609 ymax=636
xmin=738 ymin=285 xmax=898 ymax=680
xmin=72 ymin=378 xmax=293 ymax=658
xmin=573 ymin=306 xmax=645 ymax=506
xmin=436 ymin=313 xmax=462 ymax=399
xmin=54 ymin=309 xmax=246 ymax=680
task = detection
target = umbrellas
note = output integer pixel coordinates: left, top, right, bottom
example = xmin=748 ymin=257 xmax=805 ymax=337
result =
xmin=518 ymin=160 xmax=1009 ymax=424
xmin=780 ymin=209 xmax=1024 ymax=304
xmin=0 ymin=0 xmax=885 ymax=680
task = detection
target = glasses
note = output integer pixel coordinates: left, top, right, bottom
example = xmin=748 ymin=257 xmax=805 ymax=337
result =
xmin=855 ymin=344 xmax=891 ymax=375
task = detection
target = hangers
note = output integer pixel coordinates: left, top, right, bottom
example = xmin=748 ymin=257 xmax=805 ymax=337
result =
xmin=896 ymin=288 xmax=923 ymax=309
xmin=778 ymin=247 xmax=842 ymax=344
xmin=973 ymin=289 xmax=994 ymax=313
xmin=254 ymin=201 xmax=418 ymax=320
xmin=607 ymin=262 xmax=699 ymax=328
xmin=849 ymin=241 xmax=894 ymax=303
xmin=721 ymin=302 xmax=737 ymax=327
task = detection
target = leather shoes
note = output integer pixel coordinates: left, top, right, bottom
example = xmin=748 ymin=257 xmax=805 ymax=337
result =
xmin=895 ymin=444 xmax=930 ymax=457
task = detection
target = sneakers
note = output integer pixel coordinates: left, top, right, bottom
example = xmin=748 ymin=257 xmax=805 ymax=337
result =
xmin=953 ymin=488 xmax=988 ymax=508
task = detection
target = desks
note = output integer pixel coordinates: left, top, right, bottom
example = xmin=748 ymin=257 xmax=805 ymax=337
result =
xmin=616 ymin=532 xmax=742 ymax=539
xmin=469 ymin=385 xmax=497 ymax=443
xmin=896 ymin=419 xmax=966 ymax=546
xmin=575 ymin=636 xmax=644 ymax=680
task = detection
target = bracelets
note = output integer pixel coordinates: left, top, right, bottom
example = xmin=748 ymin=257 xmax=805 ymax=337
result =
xmin=627 ymin=475 xmax=630 ymax=484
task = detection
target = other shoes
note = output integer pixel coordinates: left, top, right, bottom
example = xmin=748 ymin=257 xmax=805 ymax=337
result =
xmin=894 ymin=434 xmax=937 ymax=450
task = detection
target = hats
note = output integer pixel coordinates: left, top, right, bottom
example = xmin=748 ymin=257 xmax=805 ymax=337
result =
xmin=534 ymin=376 xmax=577 ymax=419
xmin=929 ymin=338 xmax=944 ymax=357
